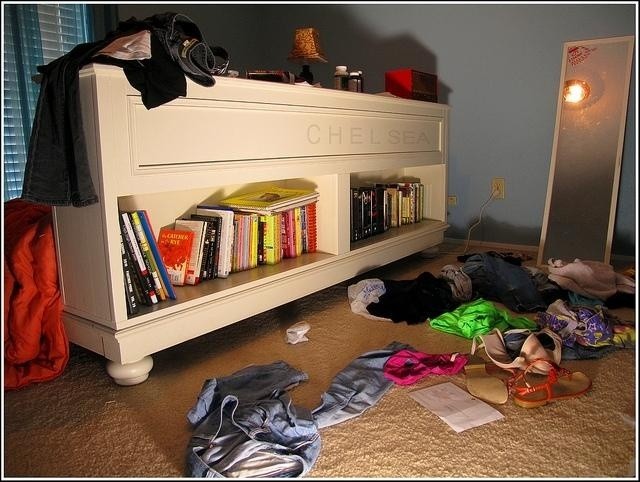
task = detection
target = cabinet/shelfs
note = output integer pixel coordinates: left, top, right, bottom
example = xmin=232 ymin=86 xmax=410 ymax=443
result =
xmin=31 ymin=63 xmax=451 ymax=386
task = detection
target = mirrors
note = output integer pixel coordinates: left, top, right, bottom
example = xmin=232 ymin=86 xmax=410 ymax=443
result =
xmin=536 ymin=35 xmax=635 ymax=269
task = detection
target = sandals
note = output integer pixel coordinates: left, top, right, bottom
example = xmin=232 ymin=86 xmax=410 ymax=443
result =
xmin=512 ymin=357 xmax=591 ymax=409
xmin=465 ymin=355 xmax=510 ymax=405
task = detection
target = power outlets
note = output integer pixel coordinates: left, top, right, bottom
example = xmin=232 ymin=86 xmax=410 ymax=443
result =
xmin=492 ymin=178 xmax=505 ymax=199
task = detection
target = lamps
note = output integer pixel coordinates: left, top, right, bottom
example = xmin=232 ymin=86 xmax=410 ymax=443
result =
xmin=563 ymin=80 xmax=590 ymax=102
xmin=286 ymin=27 xmax=328 ymax=84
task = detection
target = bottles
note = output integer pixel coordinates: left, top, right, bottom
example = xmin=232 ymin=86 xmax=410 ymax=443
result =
xmin=334 ymin=66 xmax=364 ymax=93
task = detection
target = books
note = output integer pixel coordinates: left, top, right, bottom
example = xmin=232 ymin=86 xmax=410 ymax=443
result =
xmin=119 ymin=187 xmax=319 ymax=316
xmin=350 ymin=182 xmax=424 ymax=242
